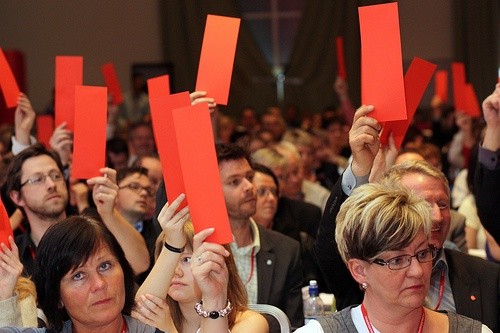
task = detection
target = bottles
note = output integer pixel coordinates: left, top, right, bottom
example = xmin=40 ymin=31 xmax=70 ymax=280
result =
xmin=305 ymin=281 xmax=324 ymax=324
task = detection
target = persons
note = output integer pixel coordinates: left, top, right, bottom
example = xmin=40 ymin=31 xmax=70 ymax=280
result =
xmin=0 ymin=141 xmax=153 ymax=294
xmin=213 ymin=142 xmax=306 ymax=332
xmin=292 ymin=173 xmax=495 ymax=332
xmin=0 ymin=214 xmax=164 ymax=332
xmin=1 ymin=74 xmax=499 ymax=258
xmin=0 ymin=235 xmax=38 ymax=329
xmin=132 ymin=193 xmax=270 ymax=332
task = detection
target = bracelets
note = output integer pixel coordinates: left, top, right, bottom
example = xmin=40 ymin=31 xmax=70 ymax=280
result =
xmin=311 ymin=102 xmax=500 ymax=333
xmin=193 ymin=298 xmax=232 ymax=320
xmin=164 ymin=241 xmax=186 ymax=253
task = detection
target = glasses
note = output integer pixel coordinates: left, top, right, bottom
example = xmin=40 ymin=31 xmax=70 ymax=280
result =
xmin=20 ymin=172 xmax=65 ymax=187
xmin=119 ymin=182 xmax=157 ymax=198
xmin=372 ymin=245 xmax=437 ymax=270
